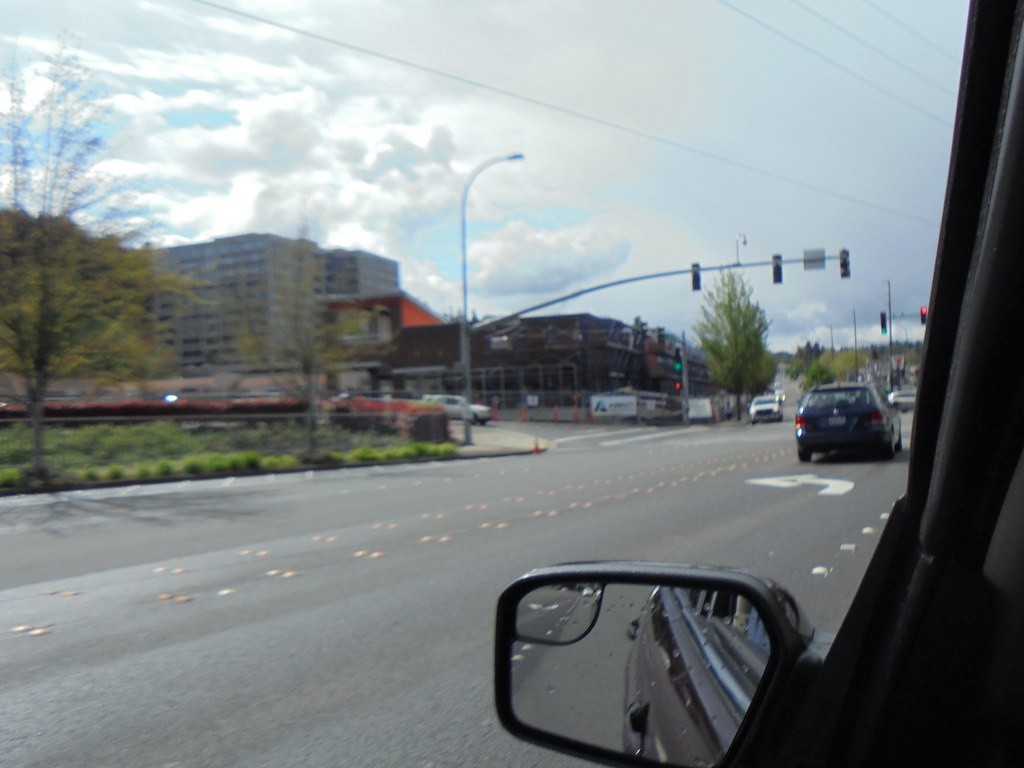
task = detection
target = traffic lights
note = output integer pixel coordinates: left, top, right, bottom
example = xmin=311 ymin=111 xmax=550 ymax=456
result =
xmin=921 ymin=307 xmax=927 ymax=325
xmin=674 ymin=382 xmax=682 ymax=390
xmin=880 ymin=313 xmax=886 ymax=334
xmin=674 ymin=348 xmax=682 ymax=371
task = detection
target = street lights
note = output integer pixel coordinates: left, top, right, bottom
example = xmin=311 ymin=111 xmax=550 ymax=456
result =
xmin=813 ymin=324 xmax=835 ymax=377
xmin=462 ymin=152 xmax=524 ymax=437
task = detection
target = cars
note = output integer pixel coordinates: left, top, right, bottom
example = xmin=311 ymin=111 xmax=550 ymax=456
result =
xmin=772 ymin=382 xmax=785 ymax=404
xmin=583 ymin=586 xmax=594 ymax=597
xmin=888 ymin=389 xmax=915 ymax=412
xmin=421 ymin=396 xmax=492 ymax=427
xmin=624 ymin=587 xmax=771 ymax=767
xmin=796 ymin=382 xmax=902 ymax=462
xmin=747 ymin=396 xmax=783 ymax=426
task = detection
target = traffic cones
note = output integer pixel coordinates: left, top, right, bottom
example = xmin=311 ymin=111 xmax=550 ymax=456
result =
xmin=533 ymin=437 xmax=541 ymax=453
